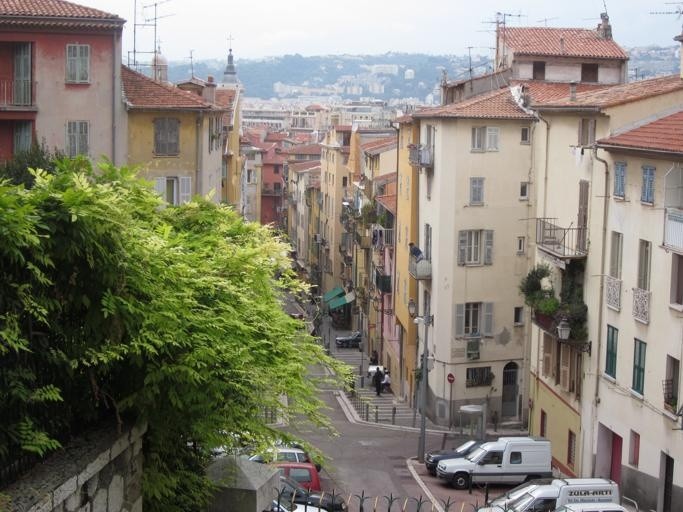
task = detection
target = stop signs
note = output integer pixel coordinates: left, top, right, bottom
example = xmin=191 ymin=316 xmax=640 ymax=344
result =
xmin=447 ymin=373 xmax=455 ymax=384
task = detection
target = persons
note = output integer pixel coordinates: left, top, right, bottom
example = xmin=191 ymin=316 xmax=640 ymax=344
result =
xmin=365 ymin=350 xmax=390 ymax=397
xmin=491 ymin=410 xmax=499 ymax=432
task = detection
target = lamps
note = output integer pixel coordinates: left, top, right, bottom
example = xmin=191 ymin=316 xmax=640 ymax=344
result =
xmin=408 ymin=298 xmax=434 ymax=328
xmin=372 ymin=296 xmax=392 ymax=316
xmin=556 ymin=316 xmax=591 ymax=357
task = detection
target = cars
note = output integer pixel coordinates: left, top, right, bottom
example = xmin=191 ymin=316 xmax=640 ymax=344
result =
xmin=335 ymin=332 xmax=361 ymax=348
xmin=426 ymin=439 xmax=498 ymax=477
xmin=289 ymin=312 xmax=306 ymax=331
xmin=187 ymin=429 xmax=348 ymax=512
xmin=478 ymin=477 xmax=629 ymax=512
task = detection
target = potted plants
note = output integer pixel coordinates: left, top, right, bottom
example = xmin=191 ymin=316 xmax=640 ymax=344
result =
xmin=525 ymin=289 xmax=559 ymax=328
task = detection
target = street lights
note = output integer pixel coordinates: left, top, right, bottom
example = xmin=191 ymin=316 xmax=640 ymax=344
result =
xmin=408 ymin=295 xmax=432 ymax=464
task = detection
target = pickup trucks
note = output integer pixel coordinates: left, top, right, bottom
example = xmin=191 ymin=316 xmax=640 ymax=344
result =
xmin=436 ymin=436 xmax=552 ymax=489
